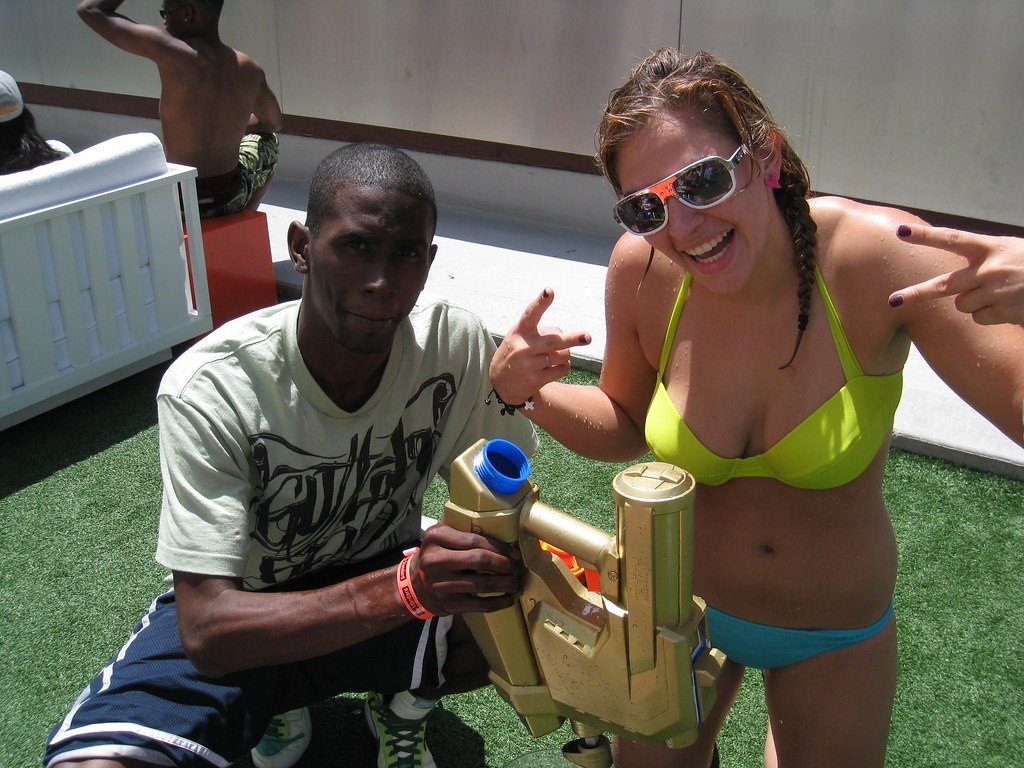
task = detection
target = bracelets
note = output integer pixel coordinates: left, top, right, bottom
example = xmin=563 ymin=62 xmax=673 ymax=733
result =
xmin=485 ymin=388 xmax=535 ymax=416
xmin=396 ymin=547 xmax=435 ymax=620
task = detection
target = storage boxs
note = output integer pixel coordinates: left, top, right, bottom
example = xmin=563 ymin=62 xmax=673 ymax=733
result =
xmin=182 ymin=211 xmax=277 ymax=342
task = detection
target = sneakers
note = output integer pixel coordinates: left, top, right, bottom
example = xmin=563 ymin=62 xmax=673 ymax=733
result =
xmin=251 ymin=707 xmax=311 ymax=768
xmin=364 ymin=691 xmax=439 ymax=768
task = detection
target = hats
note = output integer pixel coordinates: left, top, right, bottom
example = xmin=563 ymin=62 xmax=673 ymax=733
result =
xmin=0 ymin=70 xmax=23 ymax=123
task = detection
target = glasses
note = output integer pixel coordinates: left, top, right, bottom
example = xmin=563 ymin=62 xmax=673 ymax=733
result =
xmin=613 ymin=145 xmax=746 ymax=236
xmin=159 ymin=6 xmax=183 ymax=19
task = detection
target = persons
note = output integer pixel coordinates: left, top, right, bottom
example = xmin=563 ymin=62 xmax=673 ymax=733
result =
xmin=76 ymin=0 xmax=282 ymax=217
xmin=490 ymin=47 xmax=1024 ymax=768
xmin=44 ymin=143 xmax=521 ymax=768
xmin=0 ymin=69 xmax=75 ymax=176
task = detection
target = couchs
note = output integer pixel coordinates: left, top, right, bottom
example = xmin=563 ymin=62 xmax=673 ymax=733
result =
xmin=0 ymin=132 xmax=213 ymax=431
